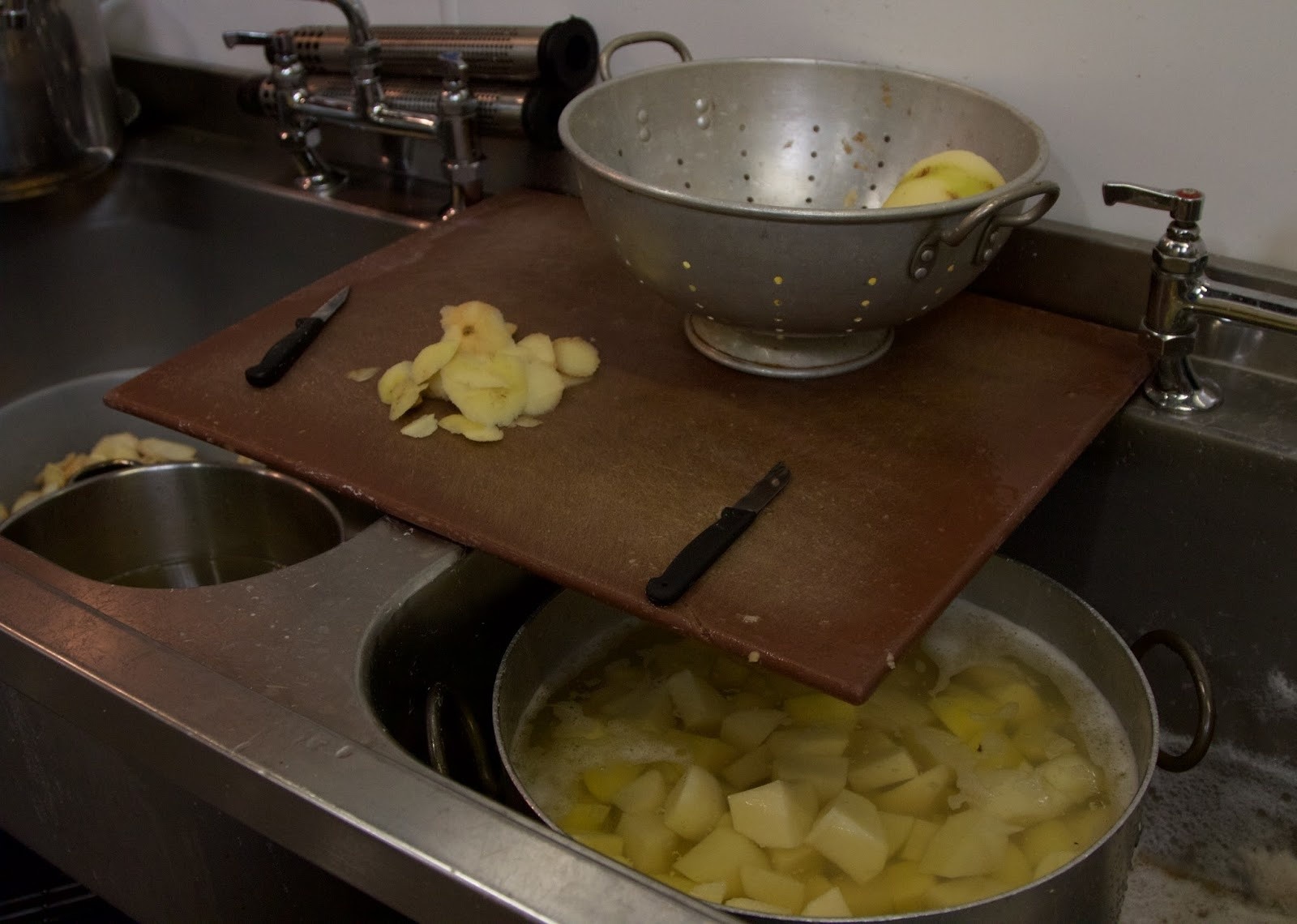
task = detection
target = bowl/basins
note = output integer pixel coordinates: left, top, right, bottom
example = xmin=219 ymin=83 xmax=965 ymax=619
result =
xmin=562 ymin=33 xmax=1058 ymax=379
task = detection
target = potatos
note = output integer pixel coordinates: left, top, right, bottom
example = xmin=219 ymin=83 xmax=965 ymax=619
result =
xmin=874 ymin=145 xmax=1011 ymax=211
xmin=538 ymin=635 xmax=1116 ymax=920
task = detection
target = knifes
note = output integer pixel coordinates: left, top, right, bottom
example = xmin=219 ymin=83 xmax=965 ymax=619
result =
xmin=246 ymin=285 xmax=351 ymax=390
xmin=647 ymin=465 xmax=796 ymax=607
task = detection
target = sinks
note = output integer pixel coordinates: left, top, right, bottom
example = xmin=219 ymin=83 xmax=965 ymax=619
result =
xmin=352 ymin=402 xmax=1295 ymax=924
xmin=2 ymin=370 xmax=417 ymax=599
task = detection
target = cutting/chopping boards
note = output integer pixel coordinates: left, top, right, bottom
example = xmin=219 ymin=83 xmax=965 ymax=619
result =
xmin=104 ymin=191 xmax=1163 ymax=709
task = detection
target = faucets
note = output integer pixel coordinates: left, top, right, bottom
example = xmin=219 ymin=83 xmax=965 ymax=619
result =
xmin=1101 ymin=179 xmax=1297 ymax=416
xmin=0 ymin=0 xmax=121 ymax=168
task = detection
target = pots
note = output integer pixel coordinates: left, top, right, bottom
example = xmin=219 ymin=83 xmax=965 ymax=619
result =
xmin=2 ymin=460 xmax=352 ymax=593
xmin=431 ymin=549 xmax=1218 ymax=924
